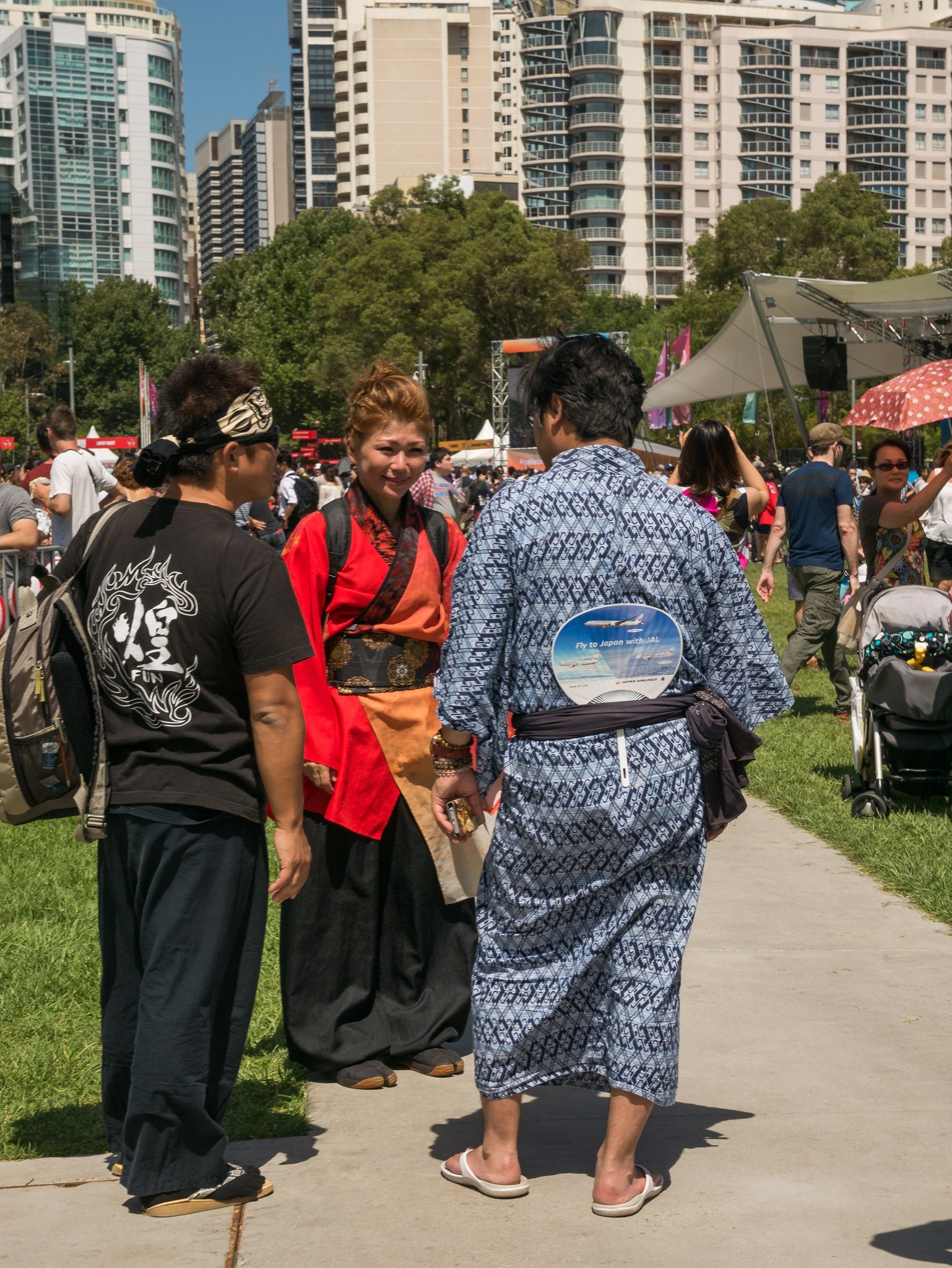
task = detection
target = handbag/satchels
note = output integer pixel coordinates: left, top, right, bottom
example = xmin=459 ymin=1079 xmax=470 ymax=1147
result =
xmin=837 ymin=585 xmax=876 ymax=654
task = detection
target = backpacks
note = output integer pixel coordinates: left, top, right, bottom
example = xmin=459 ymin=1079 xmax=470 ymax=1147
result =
xmin=285 ymin=472 xmax=319 ymax=532
xmin=0 ymin=501 xmax=133 ymax=844
xmin=458 ymin=481 xmax=476 ymax=509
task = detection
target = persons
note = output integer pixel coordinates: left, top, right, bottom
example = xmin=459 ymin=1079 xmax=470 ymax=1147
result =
xmin=279 ymin=363 xmax=466 ymax=1089
xmin=37 ymin=353 xmax=315 ymax=1219
xmin=430 ymin=328 xmax=797 ymax=1218
xmin=0 ymin=406 xmax=951 ymax=719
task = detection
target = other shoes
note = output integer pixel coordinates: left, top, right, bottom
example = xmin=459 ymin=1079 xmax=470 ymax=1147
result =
xmin=402 ymin=1047 xmax=464 ymax=1076
xmin=806 ymin=654 xmax=824 ymax=671
xmin=750 ymin=558 xmax=763 ymax=564
xmin=836 ymin=714 xmax=849 ymax=718
xmin=787 ymin=629 xmax=796 ymax=642
xmin=329 ymin=1059 xmax=397 ymax=1089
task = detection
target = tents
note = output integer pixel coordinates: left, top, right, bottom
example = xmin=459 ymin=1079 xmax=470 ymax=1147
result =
xmin=448 ymin=419 xmax=508 ymax=468
xmin=640 ymin=268 xmax=952 ymax=461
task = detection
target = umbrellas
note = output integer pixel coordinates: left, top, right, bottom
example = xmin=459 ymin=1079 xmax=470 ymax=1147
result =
xmin=840 ymin=356 xmax=952 ymax=439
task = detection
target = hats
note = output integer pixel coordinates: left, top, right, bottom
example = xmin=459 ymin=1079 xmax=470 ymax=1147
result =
xmin=809 ymin=422 xmax=853 ymax=447
xmin=665 ymin=463 xmax=674 ymax=470
xmin=314 ymin=463 xmax=321 ymax=469
xmin=857 ymin=470 xmax=871 ymax=479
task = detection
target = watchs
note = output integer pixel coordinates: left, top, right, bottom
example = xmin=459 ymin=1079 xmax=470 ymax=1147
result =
xmin=429 ymin=732 xmax=471 ymax=759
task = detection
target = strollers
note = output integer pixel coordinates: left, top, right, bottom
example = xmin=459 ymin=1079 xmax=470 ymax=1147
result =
xmin=842 ymin=584 xmax=952 ymax=819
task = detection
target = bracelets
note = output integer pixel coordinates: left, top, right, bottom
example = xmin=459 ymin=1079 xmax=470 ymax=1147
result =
xmin=433 ymin=754 xmax=473 ymax=777
xmin=437 ymin=728 xmax=473 ymax=750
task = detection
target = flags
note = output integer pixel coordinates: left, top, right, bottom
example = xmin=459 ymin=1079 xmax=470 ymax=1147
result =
xmin=649 ymin=331 xmax=669 ymax=430
xmin=671 ymin=323 xmax=691 ymax=426
xmin=743 ymin=392 xmax=757 ymax=424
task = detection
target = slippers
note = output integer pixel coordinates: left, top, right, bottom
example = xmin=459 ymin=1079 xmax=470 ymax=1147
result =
xmin=592 ymin=1163 xmax=664 ymax=1217
xmin=440 ymin=1148 xmax=530 ymax=1198
xmin=111 ymin=1162 xmax=124 ymax=1175
xmin=142 ymin=1162 xmax=274 ymax=1217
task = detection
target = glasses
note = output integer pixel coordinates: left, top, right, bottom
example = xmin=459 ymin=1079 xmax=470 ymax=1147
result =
xmin=874 ymin=461 xmax=909 ymax=471
